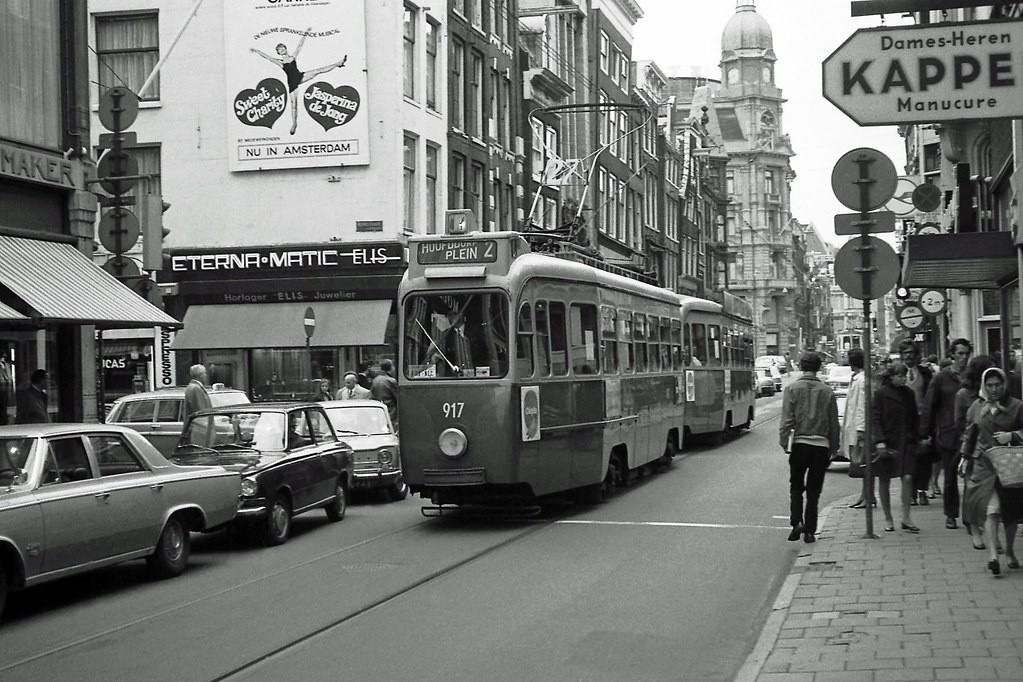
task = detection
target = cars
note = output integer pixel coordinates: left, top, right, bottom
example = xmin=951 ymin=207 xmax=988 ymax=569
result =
xmin=316 ymin=400 xmax=408 ymax=500
xmin=170 ymin=402 xmax=355 ymax=545
xmin=755 ymin=367 xmax=782 ymax=398
xmin=826 ymin=366 xmax=854 ymax=397
xmin=0 ymin=424 xmax=242 ymax=622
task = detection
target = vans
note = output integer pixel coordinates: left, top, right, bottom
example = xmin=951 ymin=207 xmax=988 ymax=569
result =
xmin=755 ymin=355 xmax=786 ymax=372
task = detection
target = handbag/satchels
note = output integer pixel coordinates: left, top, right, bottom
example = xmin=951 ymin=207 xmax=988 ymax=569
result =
xmin=985 ymin=431 xmax=1023 ymax=488
xmin=872 ymin=446 xmax=900 ymax=477
xmin=848 ymin=439 xmax=865 ymax=479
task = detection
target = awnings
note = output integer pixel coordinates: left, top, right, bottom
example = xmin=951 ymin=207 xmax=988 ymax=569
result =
xmin=169 ymin=299 xmax=396 ymax=350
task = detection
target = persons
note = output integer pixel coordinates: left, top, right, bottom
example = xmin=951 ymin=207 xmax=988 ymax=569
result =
xmin=780 ymin=353 xmax=841 ymax=543
xmin=185 ymin=364 xmax=217 ymax=449
xmin=311 ymin=359 xmax=399 ymax=434
xmin=15 ymin=369 xmax=50 ymax=424
xmin=436 ymin=305 xmax=487 ymax=377
xmin=840 ymin=337 xmax=1023 ymax=576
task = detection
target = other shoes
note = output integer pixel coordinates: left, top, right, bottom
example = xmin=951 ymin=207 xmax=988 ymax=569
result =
xmin=974 ymin=544 xmax=985 ymax=549
xmin=1007 ymin=561 xmax=1019 ymax=569
xmin=988 ymin=558 xmax=1000 ymax=574
xmin=805 ymin=533 xmax=815 ymax=543
xmin=788 ymin=522 xmax=805 ymax=541
xmin=997 ymin=546 xmax=1004 ymax=554
xmin=885 ymin=527 xmax=893 ymax=530
xmin=902 ymin=522 xmax=919 ymax=531
xmin=935 ymin=490 xmax=940 ymax=494
xmin=967 ymin=525 xmax=982 ymax=536
xmin=928 ymin=494 xmax=935 ymax=499
xmin=919 ymin=492 xmax=928 ymax=504
xmin=946 ymin=517 xmax=956 ymax=528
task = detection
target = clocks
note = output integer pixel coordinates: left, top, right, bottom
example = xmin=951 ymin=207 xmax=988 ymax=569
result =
xmin=726 ymin=67 xmax=741 ymax=85
xmin=761 ymin=67 xmax=772 ymax=85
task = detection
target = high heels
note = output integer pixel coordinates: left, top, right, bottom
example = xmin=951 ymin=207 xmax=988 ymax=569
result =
xmin=849 ymin=499 xmax=862 ymax=508
xmin=854 ymin=498 xmax=876 ymax=508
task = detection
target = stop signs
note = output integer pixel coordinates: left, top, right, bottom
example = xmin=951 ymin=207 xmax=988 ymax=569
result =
xmin=304 ymin=307 xmax=315 ymax=337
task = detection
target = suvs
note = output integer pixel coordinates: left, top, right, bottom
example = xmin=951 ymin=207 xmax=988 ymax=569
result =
xmin=101 ymin=384 xmax=259 ymax=462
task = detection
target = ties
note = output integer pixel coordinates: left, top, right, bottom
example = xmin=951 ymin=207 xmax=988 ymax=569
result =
xmin=909 ymin=370 xmax=914 ymax=380
xmin=457 ymin=329 xmax=467 ymax=370
xmin=350 ymin=391 xmax=352 ymax=395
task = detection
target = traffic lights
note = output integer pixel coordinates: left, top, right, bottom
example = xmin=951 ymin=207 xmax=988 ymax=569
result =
xmin=142 ymin=194 xmax=171 ymax=270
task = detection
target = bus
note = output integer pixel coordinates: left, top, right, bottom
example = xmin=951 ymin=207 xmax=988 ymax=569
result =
xmin=677 ymin=290 xmax=756 ymax=453
xmin=397 ymin=209 xmax=687 ymax=522
